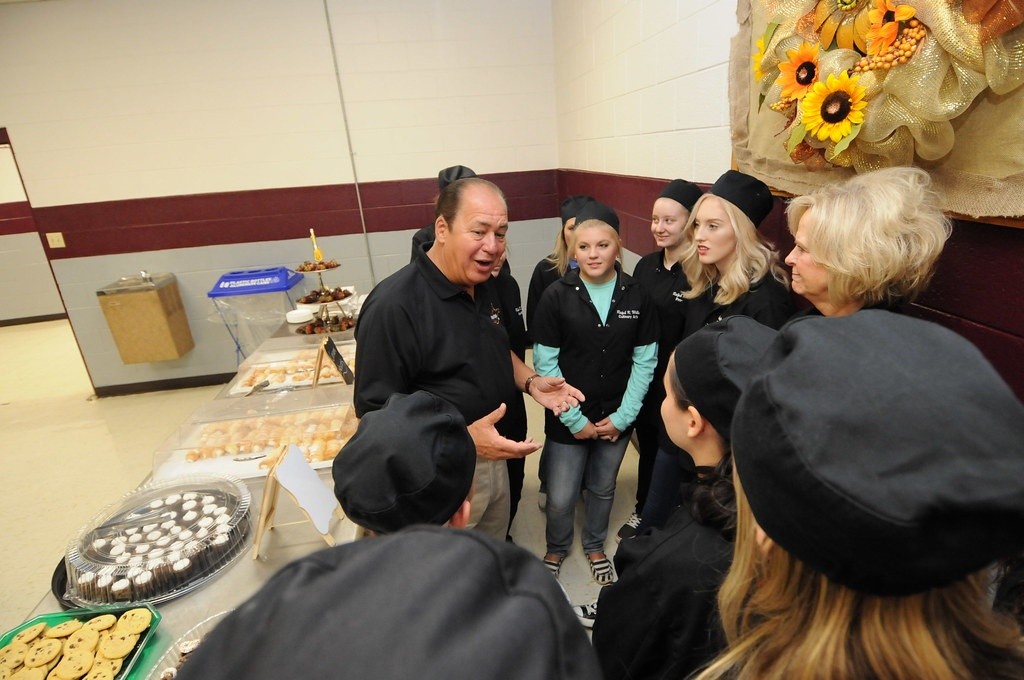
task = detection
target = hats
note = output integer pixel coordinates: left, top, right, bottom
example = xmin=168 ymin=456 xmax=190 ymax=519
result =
xmin=675 ymin=315 xmax=778 ymax=439
xmin=708 ymin=169 xmax=774 ymax=227
xmin=332 ymin=389 xmax=476 ymax=533
xmin=559 ymin=196 xmax=597 ymax=224
xmin=575 ymin=201 xmax=619 ymax=234
xmin=728 ymin=309 xmax=1023 ymax=594
xmin=660 ymin=179 xmax=703 ymax=212
xmin=170 ymin=521 xmax=605 ymax=680
xmin=438 ymin=165 xmax=476 ymax=192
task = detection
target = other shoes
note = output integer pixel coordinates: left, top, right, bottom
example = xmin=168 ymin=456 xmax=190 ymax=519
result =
xmin=542 ymin=552 xmax=564 ymax=577
xmin=585 ymin=552 xmax=613 ymax=585
xmin=538 ymin=493 xmax=546 ymax=509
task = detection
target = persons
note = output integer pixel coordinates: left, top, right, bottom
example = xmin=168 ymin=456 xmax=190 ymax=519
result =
xmin=533 ymin=202 xmax=659 ymax=585
xmin=614 ymin=180 xmax=720 ymax=544
xmin=592 ymin=314 xmax=781 ymax=680
xmin=410 ymin=166 xmax=527 ymax=552
xmin=573 ymin=170 xmax=795 ymax=629
xmin=526 ymin=194 xmax=599 ymax=512
xmin=353 ymin=178 xmax=586 ymax=561
xmin=174 ymin=390 xmax=602 ymax=680
xmin=694 ymin=310 xmax=1024 ymax=680
xmin=785 ymin=167 xmax=952 ymax=343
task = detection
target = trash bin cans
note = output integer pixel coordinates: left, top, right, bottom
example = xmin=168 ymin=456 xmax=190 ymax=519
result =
xmin=96 ymin=272 xmax=195 ymax=363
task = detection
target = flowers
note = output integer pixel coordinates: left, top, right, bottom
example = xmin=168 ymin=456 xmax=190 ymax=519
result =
xmin=753 ymin=0 xmax=918 ymax=159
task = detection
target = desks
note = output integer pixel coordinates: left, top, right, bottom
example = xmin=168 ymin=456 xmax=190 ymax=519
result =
xmin=42 ymin=291 xmax=359 ymax=680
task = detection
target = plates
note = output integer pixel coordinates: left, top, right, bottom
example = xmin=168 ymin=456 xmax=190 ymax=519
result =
xmin=294 ymin=264 xmax=340 ymax=273
xmin=285 ymin=304 xmax=321 ymax=323
xmin=296 ymin=318 xmax=357 ymax=343
xmin=296 ymin=291 xmax=358 ymax=307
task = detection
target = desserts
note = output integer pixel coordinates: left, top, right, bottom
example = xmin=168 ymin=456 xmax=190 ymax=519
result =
xmin=75 ymin=492 xmax=232 ymax=603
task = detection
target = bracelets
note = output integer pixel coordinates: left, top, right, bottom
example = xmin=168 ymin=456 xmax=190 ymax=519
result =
xmin=525 ymin=374 xmax=540 ymax=396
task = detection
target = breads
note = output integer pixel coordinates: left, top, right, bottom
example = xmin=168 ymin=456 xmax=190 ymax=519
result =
xmin=240 ymin=348 xmax=355 ymax=385
xmin=185 ymin=407 xmax=358 ymax=468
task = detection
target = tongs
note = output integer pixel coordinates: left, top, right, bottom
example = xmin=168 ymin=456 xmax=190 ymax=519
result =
xmin=94 ymin=498 xmax=171 ymax=539
xmin=242 ymin=381 xmax=289 ymax=397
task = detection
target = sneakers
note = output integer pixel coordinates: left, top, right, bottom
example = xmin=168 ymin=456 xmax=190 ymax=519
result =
xmin=615 ymin=513 xmax=642 ymax=543
xmin=573 ymin=600 xmax=597 ymax=627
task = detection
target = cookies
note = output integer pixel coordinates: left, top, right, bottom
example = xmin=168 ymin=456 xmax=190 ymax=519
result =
xmin=0 ymin=608 xmax=152 ymax=680
